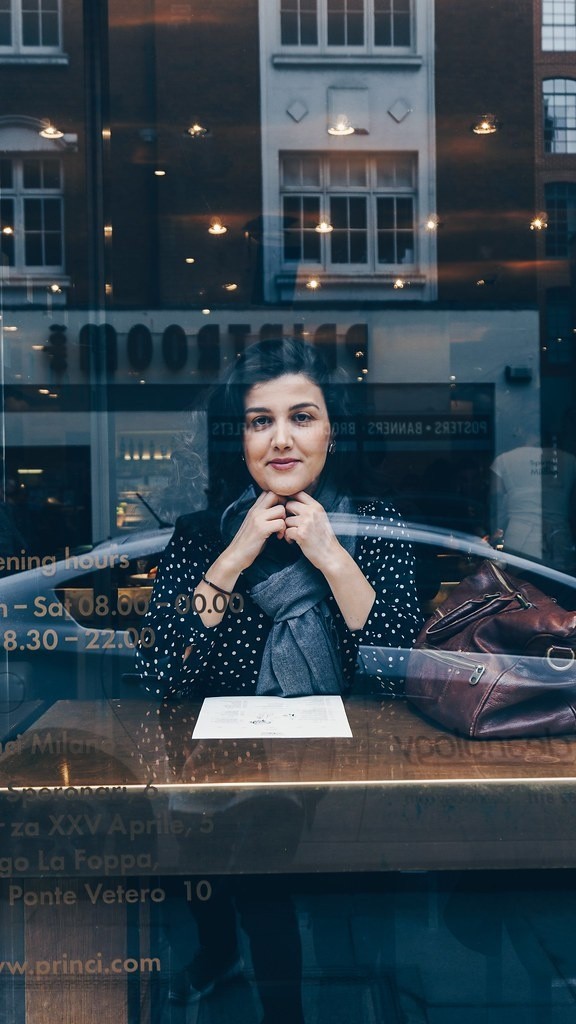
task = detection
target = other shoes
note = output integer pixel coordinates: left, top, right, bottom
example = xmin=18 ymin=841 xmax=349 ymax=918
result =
xmin=169 ymin=955 xmax=246 ymax=1003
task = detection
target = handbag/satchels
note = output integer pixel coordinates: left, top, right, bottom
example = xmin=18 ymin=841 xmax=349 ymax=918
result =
xmin=403 ymin=528 xmax=573 ymax=737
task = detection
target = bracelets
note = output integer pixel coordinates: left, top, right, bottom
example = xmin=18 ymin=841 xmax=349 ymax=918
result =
xmin=200 ymin=576 xmax=236 ymax=597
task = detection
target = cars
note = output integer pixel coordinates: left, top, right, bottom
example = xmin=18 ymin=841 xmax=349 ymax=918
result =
xmin=1 ymin=512 xmax=575 ymax=755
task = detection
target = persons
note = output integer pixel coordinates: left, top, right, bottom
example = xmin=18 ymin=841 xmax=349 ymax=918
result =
xmin=131 ymin=337 xmax=431 ymax=727
xmin=159 ymin=671 xmax=340 ymax=1023
xmin=485 ymin=399 xmax=575 ymax=579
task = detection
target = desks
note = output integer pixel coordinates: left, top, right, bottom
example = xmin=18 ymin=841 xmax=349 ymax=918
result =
xmin=0 ymin=695 xmax=576 ymax=1023
